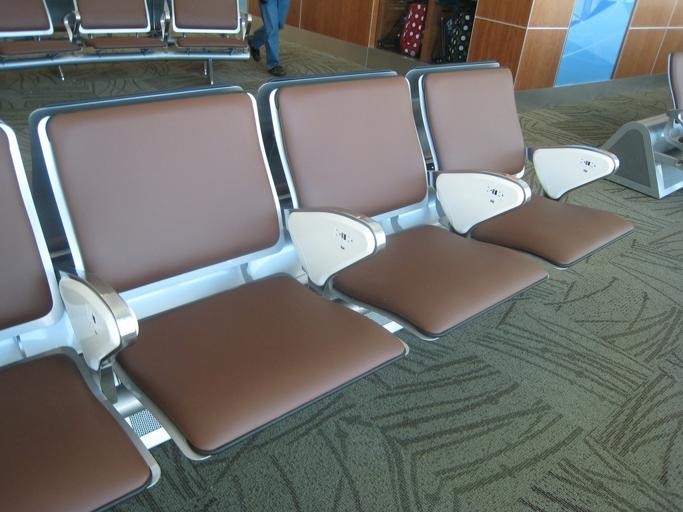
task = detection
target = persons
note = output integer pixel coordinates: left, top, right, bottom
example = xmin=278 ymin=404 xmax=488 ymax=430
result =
xmin=247 ymin=1 xmax=291 ymax=78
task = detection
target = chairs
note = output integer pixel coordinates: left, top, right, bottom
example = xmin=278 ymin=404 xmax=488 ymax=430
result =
xmin=0 ymin=120 xmax=161 ymax=511
xmin=31 ymin=86 xmax=410 ymax=461
xmin=2 ymin=2 xmax=251 ymax=86
xmin=405 ymin=60 xmax=634 ymax=270
xmin=256 ymin=69 xmax=549 ymax=342
xmin=0 ymin=0 xmax=635 ymax=512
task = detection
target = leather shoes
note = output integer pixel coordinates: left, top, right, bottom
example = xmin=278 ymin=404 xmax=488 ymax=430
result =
xmin=268 ymin=66 xmax=286 ymax=76
xmin=249 ymin=36 xmax=261 ymax=62
